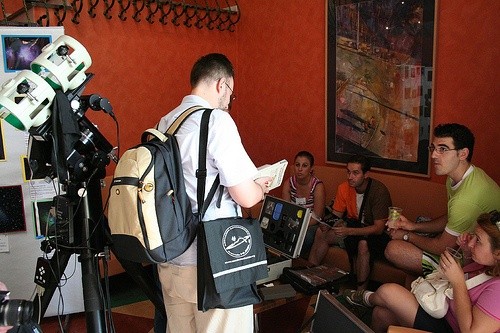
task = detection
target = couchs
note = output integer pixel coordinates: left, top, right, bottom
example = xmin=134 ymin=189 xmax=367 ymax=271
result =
xmin=293 ymin=166 xmax=448 ymax=285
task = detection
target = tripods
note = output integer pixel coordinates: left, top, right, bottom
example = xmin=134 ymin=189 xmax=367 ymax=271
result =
xmin=29 ymin=185 xmax=164 ymax=333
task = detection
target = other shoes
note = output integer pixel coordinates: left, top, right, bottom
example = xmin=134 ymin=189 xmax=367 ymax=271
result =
xmin=345 ymin=289 xmax=372 ymax=308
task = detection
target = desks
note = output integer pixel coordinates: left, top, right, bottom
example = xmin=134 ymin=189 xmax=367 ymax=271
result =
xmin=98 ymin=250 xmax=312 ymax=333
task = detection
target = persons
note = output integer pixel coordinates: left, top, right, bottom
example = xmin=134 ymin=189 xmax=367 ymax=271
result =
xmin=281 ymin=150 xmax=325 ymax=260
xmin=344 ymin=210 xmax=500 ymax=333
xmin=149 ymin=52 xmax=271 ymax=333
xmin=385 ymin=123 xmax=500 ymax=274
xmin=308 ymin=157 xmax=393 ymax=291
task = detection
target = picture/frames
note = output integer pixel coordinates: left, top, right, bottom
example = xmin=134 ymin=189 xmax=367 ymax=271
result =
xmin=324 ymin=0 xmax=439 ymax=178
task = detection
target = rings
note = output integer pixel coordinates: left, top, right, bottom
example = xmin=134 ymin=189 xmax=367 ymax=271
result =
xmin=442 ymin=265 xmax=445 ymax=268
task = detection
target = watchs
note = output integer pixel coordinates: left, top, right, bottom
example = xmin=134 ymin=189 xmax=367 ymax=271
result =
xmin=403 ymin=231 xmax=410 ymax=241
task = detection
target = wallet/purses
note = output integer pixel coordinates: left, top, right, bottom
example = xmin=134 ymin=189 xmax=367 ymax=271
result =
xmin=258 ymin=283 xmax=296 ymax=300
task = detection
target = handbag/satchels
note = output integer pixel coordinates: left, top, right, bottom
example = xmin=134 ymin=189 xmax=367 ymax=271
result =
xmin=196 ymin=217 xmax=267 ymax=311
xmin=410 ymin=269 xmax=452 ymax=318
xmin=278 ymin=263 xmax=352 ymax=297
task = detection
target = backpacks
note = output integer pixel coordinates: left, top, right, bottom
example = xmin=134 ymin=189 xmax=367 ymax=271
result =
xmin=107 ymin=105 xmax=221 ymax=265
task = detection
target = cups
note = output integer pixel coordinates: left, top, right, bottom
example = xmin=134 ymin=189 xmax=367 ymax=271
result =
xmin=388 ymin=207 xmax=403 ymax=228
xmin=439 ymin=247 xmax=462 ymax=276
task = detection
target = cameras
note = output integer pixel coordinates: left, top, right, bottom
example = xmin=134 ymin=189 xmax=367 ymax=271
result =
xmin=0 ymin=291 xmax=33 ymax=327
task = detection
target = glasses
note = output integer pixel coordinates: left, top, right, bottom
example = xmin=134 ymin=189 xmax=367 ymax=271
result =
xmin=489 ymin=210 xmax=500 ymax=231
xmin=225 ymin=82 xmax=238 ymax=102
xmin=427 ymin=145 xmax=457 ymax=154
